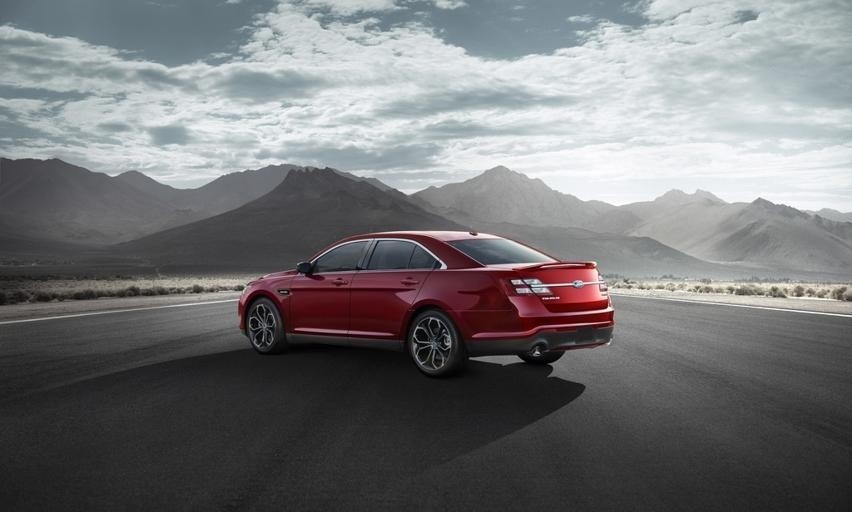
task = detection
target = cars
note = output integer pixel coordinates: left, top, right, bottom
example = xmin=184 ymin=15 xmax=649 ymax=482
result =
xmin=237 ymin=230 xmax=614 ymax=377
